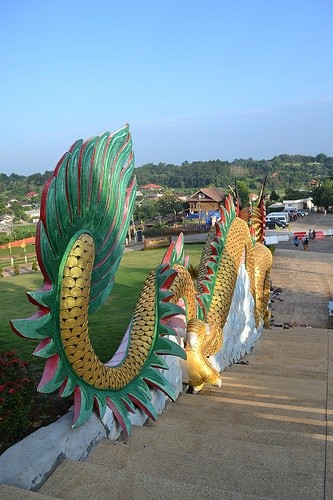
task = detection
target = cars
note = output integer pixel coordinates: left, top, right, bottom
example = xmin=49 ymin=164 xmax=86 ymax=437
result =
xmin=264 ymin=207 xmax=309 ymax=230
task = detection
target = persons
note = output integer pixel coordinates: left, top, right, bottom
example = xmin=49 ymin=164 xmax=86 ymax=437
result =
xmin=302 ymin=211 xmax=308 ymax=219
xmin=294 ymin=236 xmax=299 ymax=247
xmin=308 ymin=229 xmax=316 ymax=242
xmin=302 ymin=236 xmax=309 ymax=251
xmin=293 ymin=213 xmax=297 ymax=221
